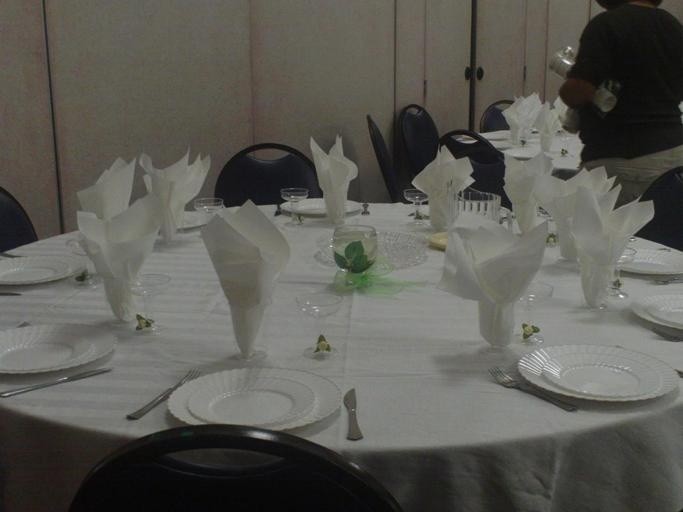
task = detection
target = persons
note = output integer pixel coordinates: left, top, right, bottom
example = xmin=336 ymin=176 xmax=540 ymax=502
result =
xmin=558 ymin=0 xmax=683 ymax=163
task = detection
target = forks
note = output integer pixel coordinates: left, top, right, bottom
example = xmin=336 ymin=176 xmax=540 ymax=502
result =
xmin=487 ymin=365 xmax=577 ymax=412
xmin=127 ymin=369 xmax=201 ymax=420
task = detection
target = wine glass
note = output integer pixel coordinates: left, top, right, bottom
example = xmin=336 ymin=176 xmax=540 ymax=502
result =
xmin=514 ymin=282 xmax=554 ymax=345
xmin=404 ymin=189 xmax=430 ymax=230
xmin=66 ymin=239 xmax=98 ymax=287
xmin=280 ymin=187 xmax=309 ymax=225
xmin=295 ymin=291 xmax=344 ymax=360
xmin=537 ymin=209 xmax=560 ymax=247
xmin=606 ymin=248 xmax=638 ymax=302
xmin=123 ymin=273 xmax=171 ymax=336
xmin=333 ymin=224 xmax=379 ymax=271
xmin=193 ymin=198 xmax=223 ymax=215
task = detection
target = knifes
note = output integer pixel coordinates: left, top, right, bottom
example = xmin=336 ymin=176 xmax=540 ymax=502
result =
xmin=0 ymin=292 xmax=23 ymax=296
xmin=0 ymin=366 xmax=114 ymax=398
xmin=344 ymin=388 xmax=363 ymax=441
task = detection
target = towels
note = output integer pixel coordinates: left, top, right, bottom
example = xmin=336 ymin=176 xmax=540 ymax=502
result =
xmin=307 ymin=134 xmax=359 ymax=227
xmin=199 ymin=197 xmax=292 ymax=362
xmin=73 ymin=147 xmax=212 ymax=332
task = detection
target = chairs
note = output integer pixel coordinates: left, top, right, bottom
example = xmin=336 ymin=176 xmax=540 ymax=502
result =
xmin=365 ymin=92 xmax=588 ymax=205
xmin=213 ymin=145 xmax=323 ymax=214
xmin=637 ymin=167 xmax=682 ymax=251
xmin=68 ymin=425 xmax=402 ymax=511
xmin=1 ymin=191 xmax=38 ymax=251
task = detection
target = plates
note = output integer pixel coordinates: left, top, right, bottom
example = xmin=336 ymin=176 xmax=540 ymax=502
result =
xmin=316 ymin=229 xmax=430 ymax=267
xmin=630 ymin=293 xmax=682 ymax=329
xmin=0 ymin=256 xmax=74 ymax=286
xmin=280 ymin=197 xmax=362 ymax=216
xmin=504 ymin=148 xmax=539 ymax=159
xmin=516 ymin=345 xmax=680 ymax=403
xmin=0 ymin=322 xmax=117 ymax=378
xmin=167 ymin=368 xmax=341 ymax=430
xmin=170 ymin=210 xmax=210 ymax=230
xmin=621 ymin=248 xmax=683 ymax=274
